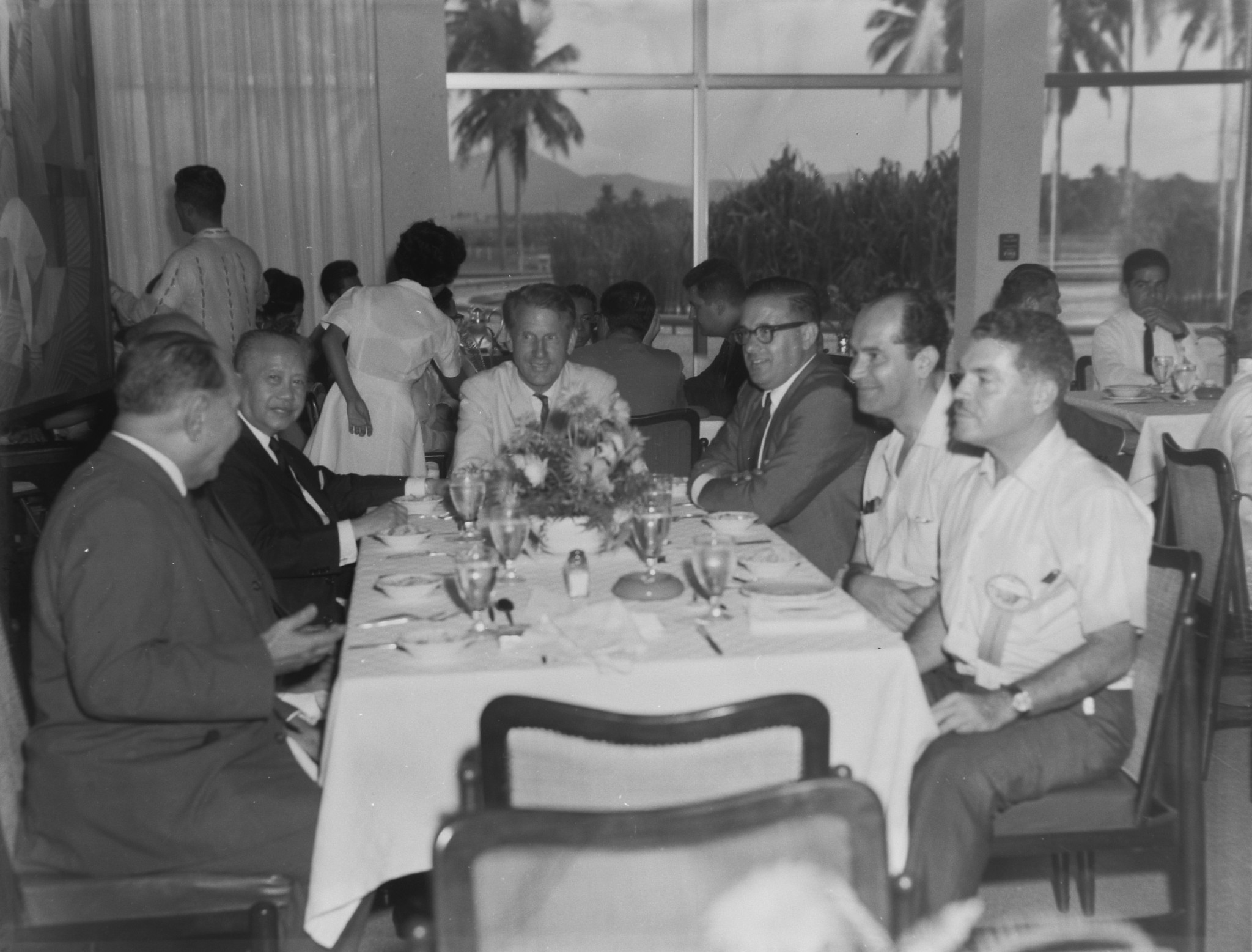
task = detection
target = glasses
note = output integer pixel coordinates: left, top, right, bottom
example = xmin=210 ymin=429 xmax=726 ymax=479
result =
xmin=577 ymin=312 xmax=595 ymax=323
xmin=733 ymin=321 xmax=810 ymax=345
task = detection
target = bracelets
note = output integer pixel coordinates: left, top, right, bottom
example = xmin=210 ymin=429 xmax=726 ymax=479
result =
xmin=843 ymin=569 xmax=862 ymax=592
xmin=1174 ymin=328 xmax=1189 ymax=339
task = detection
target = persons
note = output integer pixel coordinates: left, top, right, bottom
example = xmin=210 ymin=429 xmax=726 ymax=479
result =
xmin=214 ymin=330 xmax=445 ymax=629
xmin=679 ymin=262 xmax=985 ymax=632
xmin=1092 ymin=249 xmax=1206 ymax=390
xmin=12 ymin=331 xmax=375 ymax=952
xmin=903 ymin=309 xmax=1157 ymax=918
xmin=109 ymin=165 xmax=269 ymax=365
xmin=265 ymin=219 xmax=467 ymax=476
xmin=1191 ymin=290 xmax=1252 ymax=604
xmin=1000 ymin=263 xmax=1062 ymax=318
xmin=570 ymin=280 xmax=686 ymax=476
xmin=447 ymin=283 xmax=631 ymax=477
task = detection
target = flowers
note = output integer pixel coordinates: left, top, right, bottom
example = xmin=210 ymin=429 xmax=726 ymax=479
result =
xmin=481 ymin=382 xmax=651 ymax=539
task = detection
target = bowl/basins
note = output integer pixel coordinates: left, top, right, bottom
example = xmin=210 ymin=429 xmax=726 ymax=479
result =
xmin=1105 ymin=384 xmax=1148 ymax=398
xmin=703 ymin=511 xmax=758 ymax=535
xmin=395 ymin=630 xmax=471 ymax=658
xmin=374 ymin=526 xmax=432 ymax=548
xmin=393 ymin=496 xmax=445 ymax=516
xmin=738 ymin=554 xmax=802 ymax=577
xmin=376 ymin=572 xmax=444 ymax=601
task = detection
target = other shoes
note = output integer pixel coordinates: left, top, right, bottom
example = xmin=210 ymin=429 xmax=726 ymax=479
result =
xmin=378 ymin=887 xmax=395 ymax=907
xmin=392 ymin=902 xmax=434 ymax=936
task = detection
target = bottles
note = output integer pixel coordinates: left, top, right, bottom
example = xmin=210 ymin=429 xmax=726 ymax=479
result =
xmin=464 ymin=308 xmax=493 ymax=353
xmin=565 ymin=550 xmax=591 ymax=598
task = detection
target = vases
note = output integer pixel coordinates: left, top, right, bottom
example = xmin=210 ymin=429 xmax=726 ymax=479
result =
xmin=528 ymin=514 xmax=610 ymax=554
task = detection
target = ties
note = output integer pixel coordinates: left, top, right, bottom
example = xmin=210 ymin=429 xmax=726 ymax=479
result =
xmin=268 ymin=435 xmax=303 ymax=499
xmin=1143 ymin=322 xmax=1155 ymax=376
xmin=533 ymin=393 xmax=549 ymax=434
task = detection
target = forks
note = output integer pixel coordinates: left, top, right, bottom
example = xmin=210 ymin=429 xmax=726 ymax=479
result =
xmin=357 ymin=608 xmax=461 ymax=628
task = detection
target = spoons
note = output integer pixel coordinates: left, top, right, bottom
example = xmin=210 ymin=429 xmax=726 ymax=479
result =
xmin=496 ymin=597 xmax=515 ymax=626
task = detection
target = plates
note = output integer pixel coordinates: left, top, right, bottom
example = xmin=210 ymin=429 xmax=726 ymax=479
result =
xmin=1099 ymin=393 xmax=1154 ymax=401
xmin=740 ymin=582 xmax=836 ymax=602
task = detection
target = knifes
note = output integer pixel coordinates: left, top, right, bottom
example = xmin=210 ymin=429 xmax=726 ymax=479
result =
xmin=698 ymin=625 xmax=723 ymax=655
xmin=1114 ymin=401 xmax=1166 ymax=404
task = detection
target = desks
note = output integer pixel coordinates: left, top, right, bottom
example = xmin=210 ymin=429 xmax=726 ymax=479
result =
xmin=1061 ymin=389 xmax=1219 ymax=505
xmin=303 ymin=494 xmax=944 ymax=952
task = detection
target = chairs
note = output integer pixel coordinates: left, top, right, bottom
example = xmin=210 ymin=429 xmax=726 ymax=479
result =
xmin=394 ymin=357 xmax=1252 ymax=952
xmin=0 ymin=435 xmax=309 ymax=952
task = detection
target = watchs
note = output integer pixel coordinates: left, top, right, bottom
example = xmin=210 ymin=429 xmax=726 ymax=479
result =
xmin=1009 ymin=682 xmax=1032 ymax=719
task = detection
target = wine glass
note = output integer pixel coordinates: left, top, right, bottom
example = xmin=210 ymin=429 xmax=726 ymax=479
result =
xmin=448 ymin=473 xmax=487 ymax=540
xmin=1152 ymin=356 xmax=1173 ymax=394
xmin=452 ymin=546 xmax=498 ymax=636
xmin=612 ymin=471 xmax=685 ymax=601
xmin=1173 ymin=364 xmax=1197 ymax=406
xmin=691 ymin=534 xmax=734 ymax=622
xmin=489 ymin=504 xmax=529 ymax=582
